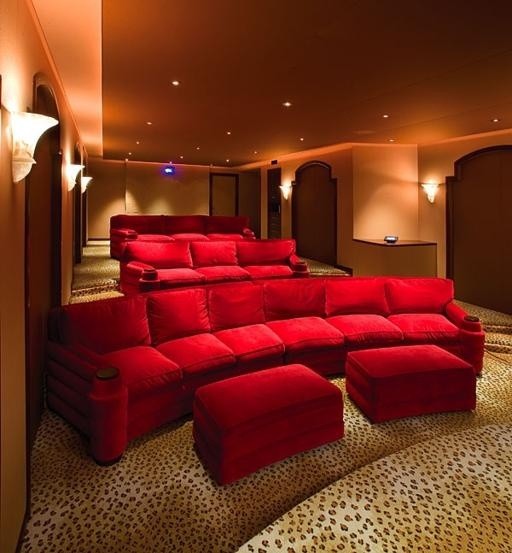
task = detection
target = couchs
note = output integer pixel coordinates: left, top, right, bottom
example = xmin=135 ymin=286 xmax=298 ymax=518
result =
xmin=109 ymin=213 xmax=257 ymax=260
xmin=116 ymin=238 xmax=310 ymax=295
xmin=43 ymin=276 xmax=484 ymax=466
xmin=190 ymin=364 xmax=348 ymax=485
xmin=344 ymin=343 xmax=478 ymax=423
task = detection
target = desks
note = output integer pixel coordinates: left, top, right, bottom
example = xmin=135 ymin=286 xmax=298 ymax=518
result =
xmin=354 ymin=237 xmax=438 ymax=276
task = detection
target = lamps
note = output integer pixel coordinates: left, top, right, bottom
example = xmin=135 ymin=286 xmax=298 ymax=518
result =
xmin=66 ymin=164 xmax=85 ymax=190
xmin=276 ymin=184 xmax=290 ymax=200
xmin=81 ymin=176 xmax=94 ymax=192
xmin=420 ymin=183 xmax=439 ymax=202
xmin=10 ymin=110 xmax=58 ymax=182
